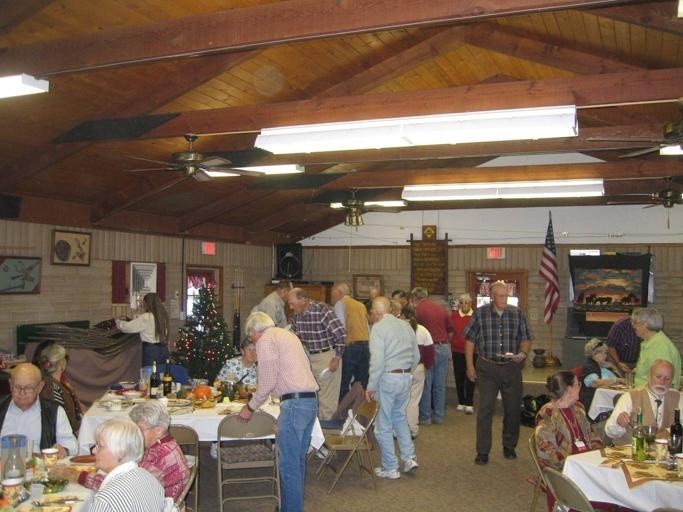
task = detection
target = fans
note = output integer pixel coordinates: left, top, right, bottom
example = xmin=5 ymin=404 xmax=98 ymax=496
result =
xmin=607 ymin=174 xmax=682 ymax=210
xmin=585 ymin=97 xmax=682 ymax=161
xmin=126 ymin=136 xmax=266 ymax=185
xmin=305 ymin=188 xmax=401 ymax=227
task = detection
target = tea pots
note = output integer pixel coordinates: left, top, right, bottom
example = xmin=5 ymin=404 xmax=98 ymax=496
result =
xmin=0 ymin=434 xmax=33 ymax=477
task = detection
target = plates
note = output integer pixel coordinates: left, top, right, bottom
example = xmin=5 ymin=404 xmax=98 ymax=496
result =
xmin=496 ymin=351 xmax=519 ymax=359
xmin=101 ymin=380 xmax=280 ymax=415
xmin=0 ymin=454 xmax=101 ymax=511
xmin=319 ymin=367 xmax=334 ymax=380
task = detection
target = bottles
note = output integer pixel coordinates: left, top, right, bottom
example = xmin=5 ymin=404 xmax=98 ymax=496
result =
xmin=531 ymin=348 xmax=545 ymax=367
xmin=150 ymin=357 xmax=172 ymax=399
xmin=632 ymin=412 xmax=647 ymax=462
xmin=669 ymin=408 xmax=682 ymax=454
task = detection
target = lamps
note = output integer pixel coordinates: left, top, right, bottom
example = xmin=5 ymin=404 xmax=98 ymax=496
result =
xmin=401 ymin=177 xmax=605 ymax=202
xmin=0 ymin=69 xmax=49 ymax=99
xmin=254 ymin=105 xmax=581 ymax=155
xmin=197 ymin=163 xmax=307 ymax=181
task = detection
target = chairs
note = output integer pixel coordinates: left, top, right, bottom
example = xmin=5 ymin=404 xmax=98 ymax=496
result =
xmin=306 ymin=381 xmax=377 ymax=495
xmin=169 ymin=423 xmax=198 ymax=511
xmin=523 ymin=366 xmax=681 ymax=512
xmin=217 ymin=410 xmax=280 ymax=511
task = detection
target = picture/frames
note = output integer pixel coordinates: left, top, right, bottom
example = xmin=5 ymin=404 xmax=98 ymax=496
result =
xmin=0 ymin=255 xmax=42 ymax=296
xmin=351 ymin=273 xmax=384 ymax=301
xmin=49 ymin=229 xmax=91 ymax=266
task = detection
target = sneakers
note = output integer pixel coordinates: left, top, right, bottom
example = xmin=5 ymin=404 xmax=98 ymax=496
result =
xmin=464 ymin=405 xmax=474 ymax=414
xmin=474 ymin=451 xmax=490 ymax=465
xmin=374 ymin=466 xmax=402 ymax=480
xmin=456 ymin=403 xmax=466 ymax=412
xmin=502 ymin=445 xmax=519 ymax=461
xmin=402 ymin=459 xmax=420 ymax=476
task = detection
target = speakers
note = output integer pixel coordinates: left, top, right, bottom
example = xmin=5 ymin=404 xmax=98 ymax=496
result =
xmin=277 ymin=243 xmax=302 ymax=279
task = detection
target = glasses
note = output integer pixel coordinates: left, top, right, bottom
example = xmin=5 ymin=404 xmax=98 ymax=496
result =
xmin=10 ymin=381 xmax=42 ymax=396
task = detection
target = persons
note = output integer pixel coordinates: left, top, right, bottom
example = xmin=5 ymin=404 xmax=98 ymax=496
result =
xmin=534 ymin=307 xmax=683 ymax=512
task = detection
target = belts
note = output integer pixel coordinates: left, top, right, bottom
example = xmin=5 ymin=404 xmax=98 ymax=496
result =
xmin=383 ymin=368 xmax=413 ymax=375
xmin=280 ymin=391 xmax=317 ymax=402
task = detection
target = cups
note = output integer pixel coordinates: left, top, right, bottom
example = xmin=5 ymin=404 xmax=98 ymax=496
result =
xmin=41 ymin=446 xmax=58 ymax=470
xmin=624 ymin=371 xmax=632 ymax=387
xmin=654 ymin=438 xmax=668 ymax=461
xmin=674 ymin=452 xmax=683 ymax=467
xmin=642 ymin=426 xmax=657 ymax=464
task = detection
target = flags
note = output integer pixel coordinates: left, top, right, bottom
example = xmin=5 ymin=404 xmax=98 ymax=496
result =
xmin=539 ymin=215 xmax=560 ymax=326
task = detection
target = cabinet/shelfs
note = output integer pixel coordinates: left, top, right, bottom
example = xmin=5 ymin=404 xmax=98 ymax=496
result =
xmin=263 ymin=282 xmax=331 ymax=303
